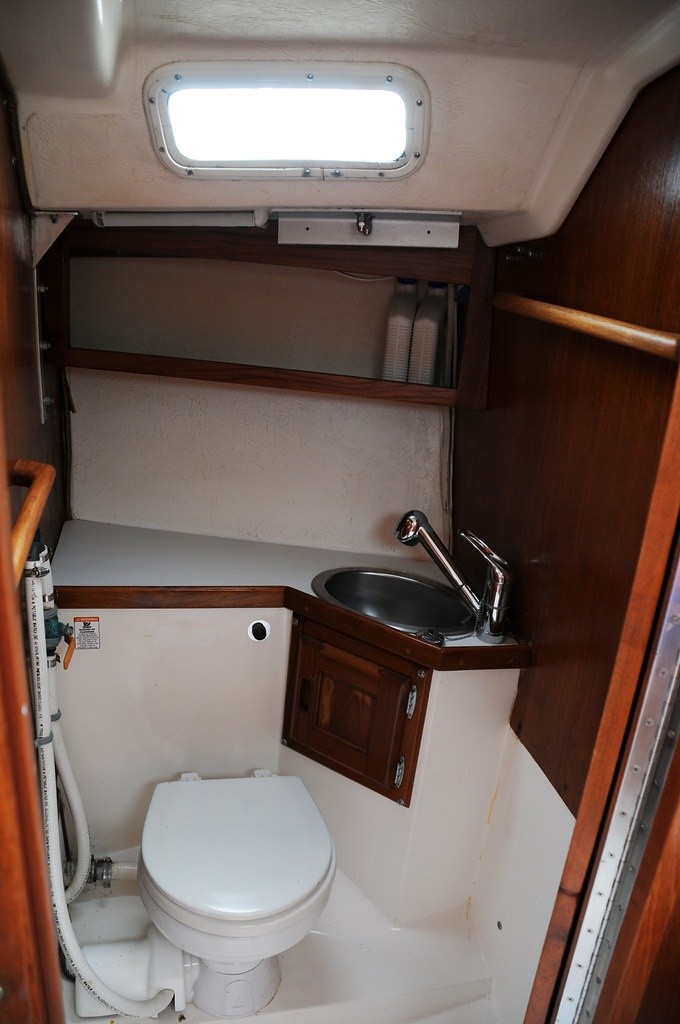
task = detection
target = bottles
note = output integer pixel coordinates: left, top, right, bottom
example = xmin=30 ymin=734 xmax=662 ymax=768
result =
xmin=408 ymin=280 xmax=447 ymax=386
xmin=382 ymin=278 xmax=417 ymax=382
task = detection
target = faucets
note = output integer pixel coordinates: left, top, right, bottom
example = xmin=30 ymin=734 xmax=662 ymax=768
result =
xmin=394 ymin=509 xmax=513 ymax=645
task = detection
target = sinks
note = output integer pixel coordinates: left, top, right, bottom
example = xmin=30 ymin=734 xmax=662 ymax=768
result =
xmin=311 ymin=567 xmax=484 ymax=636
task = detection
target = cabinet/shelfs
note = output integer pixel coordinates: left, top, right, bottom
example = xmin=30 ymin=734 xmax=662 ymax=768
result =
xmin=278 ymin=616 xmax=430 ymax=808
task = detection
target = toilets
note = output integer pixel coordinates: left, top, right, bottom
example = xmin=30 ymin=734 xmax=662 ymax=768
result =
xmin=138 ymin=768 xmax=336 ymax=1019
